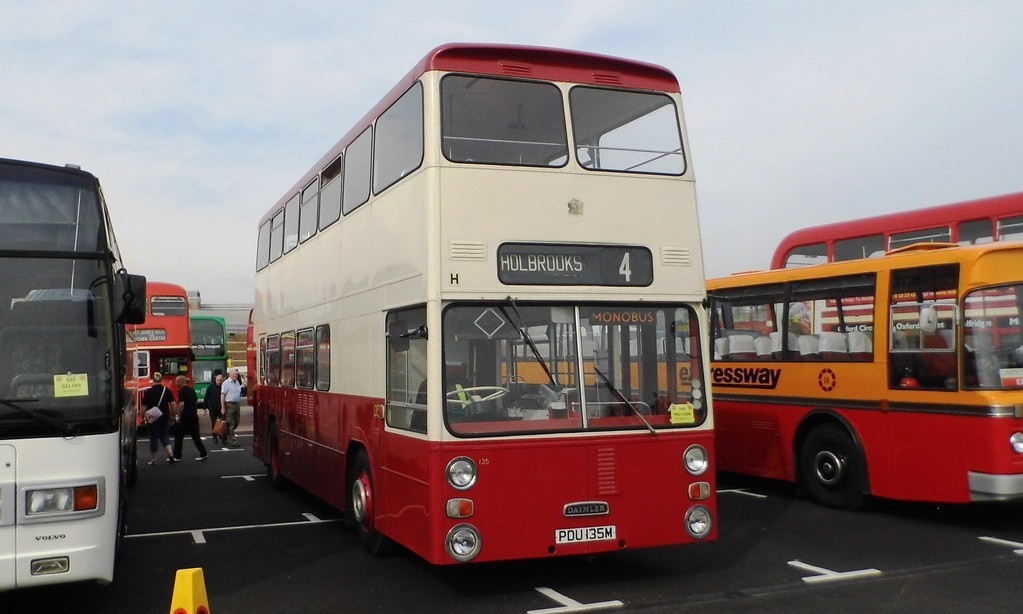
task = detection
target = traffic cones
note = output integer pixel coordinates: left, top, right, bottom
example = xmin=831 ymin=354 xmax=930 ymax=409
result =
xmin=169 ymin=568 xmax=210 ymax=614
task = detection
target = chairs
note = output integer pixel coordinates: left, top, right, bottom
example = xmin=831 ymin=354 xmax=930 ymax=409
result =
xmin=628 ymin=330 xmax=909 ymax=364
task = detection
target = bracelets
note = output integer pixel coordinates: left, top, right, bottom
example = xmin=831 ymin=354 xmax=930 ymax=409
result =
xmin=175 ymin=415 xmax=180 ymax=420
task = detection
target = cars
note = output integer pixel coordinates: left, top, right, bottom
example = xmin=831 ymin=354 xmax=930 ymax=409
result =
xmin=229 ymin=365 xmax=249 ymax=398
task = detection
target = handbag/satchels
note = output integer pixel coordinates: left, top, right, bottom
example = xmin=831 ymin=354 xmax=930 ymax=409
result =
xmin=145 ymin=406 xmax=163 ymax=424
xmin=211 ymin=414 xmax=227 ymax=437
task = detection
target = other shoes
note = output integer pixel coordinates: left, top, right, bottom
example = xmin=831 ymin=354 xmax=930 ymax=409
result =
xmin=196 ymin=455 xmax=209 ymax=461
xmin=226 ymin=442 xmax=240 ymax=447
xmin=170 ymin=453 xmax=174 ymax=463
xmin=151 ymin=459 xmax=156 ymax=464
xmin=167 ymin=457 xmax=182 ymax=462
xmin=221 ymin=438 xmax=228 ymax=445
xmin=212 ymin=436 xmax=217 ymax=443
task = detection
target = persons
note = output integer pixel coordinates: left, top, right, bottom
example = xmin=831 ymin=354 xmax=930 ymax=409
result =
xmin=220 ymin=367 xmax=242 ymax=447
xmin=165 ymin=374 xmax=209 ymax=461
xmin=235 ymin=369 xmax=248 ymax=397
xmin=202 ymin=374 xmax=226 ymax=445
xmin=140 ymin=371 xmax=178 ymax=466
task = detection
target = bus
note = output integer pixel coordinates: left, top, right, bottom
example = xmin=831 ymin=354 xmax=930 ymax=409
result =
xmin=123 ymin=279 xmax=195 ymax=428
xmin=761 ymin=187 xmax=1023 ymax=390
xmin=0 ymin=154 xmax=147 ymax=597
xmin=186 ymin=315 xmax=229 ymax=408
xmin=251 ymin=40 xmax=723 ymax=570
xmin=498 ymin=240 xmax=1022 ymax=516
